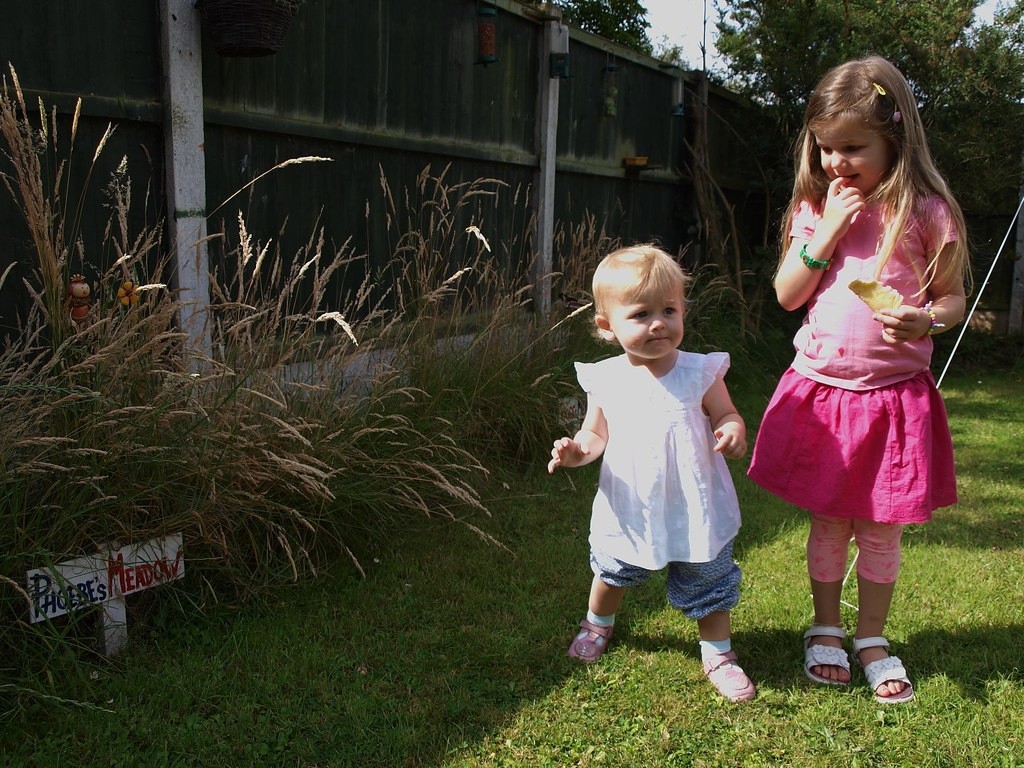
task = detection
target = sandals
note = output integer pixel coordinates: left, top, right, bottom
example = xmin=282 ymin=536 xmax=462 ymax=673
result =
xmin=804 ymin=622 xmax=851 ymax=685
xmin=852 ymin=635 xmax=914 ymax=704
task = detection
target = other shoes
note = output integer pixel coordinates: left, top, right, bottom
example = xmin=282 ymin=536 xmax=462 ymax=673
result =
xmin=569 ymin=617 xmax=613 ymax=662
xmin=705 ymin=649 xmax=756 ymax=703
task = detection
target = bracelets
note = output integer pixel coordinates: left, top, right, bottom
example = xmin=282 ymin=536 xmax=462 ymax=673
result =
xmin=918 ymin=301 xmax=945 ymax=341
xmin=799 ymin=243 xmax=832 ymax=271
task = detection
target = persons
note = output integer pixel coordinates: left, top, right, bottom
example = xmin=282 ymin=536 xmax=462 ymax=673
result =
xmin=547 ymin=246 xmax=757 ymax=704
xmin=747 ymin=56 xmax=974 ymax=704
